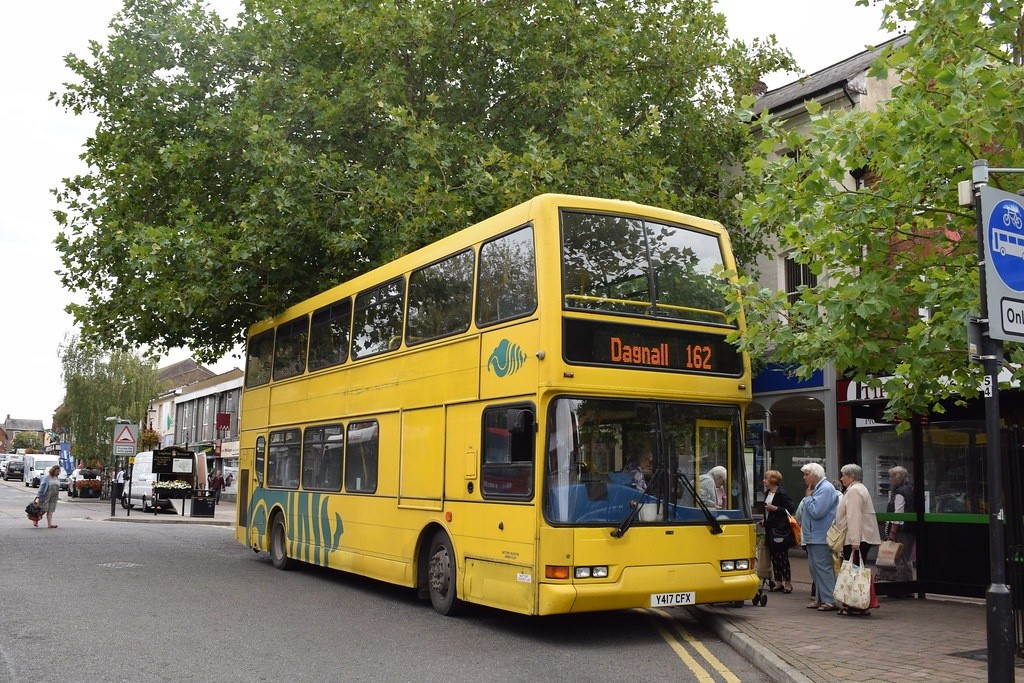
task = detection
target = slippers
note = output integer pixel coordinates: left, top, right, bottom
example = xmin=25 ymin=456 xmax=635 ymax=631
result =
xmin=836 ymin=610 xmax=853 ymax=616
xmin=861 ymin=612 xmax=871 ymax=616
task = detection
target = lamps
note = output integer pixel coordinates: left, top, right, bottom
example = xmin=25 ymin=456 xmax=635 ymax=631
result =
xmin=956 ymin=490 xmax=969 ymax=513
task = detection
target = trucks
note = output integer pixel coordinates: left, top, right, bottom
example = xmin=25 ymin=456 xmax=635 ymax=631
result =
xmin=60 ymin=443 xmax=75 ymax=475
xmin=24 ymin=454 xmax=60 ymax=488
xmin=16 ymin=449 xmax=27 ymax=454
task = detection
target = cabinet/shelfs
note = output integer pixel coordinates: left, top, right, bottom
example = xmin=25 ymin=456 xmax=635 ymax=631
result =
xmin=876 ymin=454 xmax=913 ymax=496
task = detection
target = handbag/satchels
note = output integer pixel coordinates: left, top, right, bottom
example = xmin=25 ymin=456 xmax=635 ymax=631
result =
xmin=836 ymin=551 xmax=880 ymax=609
xmin=875 ymin=539 xmax=904 ymax=581
xmin=826 ymin=518 xmax=847 ymax=551
xmin=833 ymin=548 xmax=870 ymax=609
xmin=28 ymin=498 xmax=45 ymax=521
xmin=833 ymin=552 xmax=842 ymax=574
xmin=875 ymin=541 xmax=904 ymax=570
xmin=25 ymin=502 xmax=40 ymax=516
xmin=785 ymin=508 xmax=802 ymax=544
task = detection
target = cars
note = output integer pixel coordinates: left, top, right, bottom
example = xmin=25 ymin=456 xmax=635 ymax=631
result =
xmin=0 ymin=454 xmax=24 ymax=481
xmin=40 ymin=467 xmax=71 ymax=491
xmin=67 ymin=469 xmax=102 ymax=498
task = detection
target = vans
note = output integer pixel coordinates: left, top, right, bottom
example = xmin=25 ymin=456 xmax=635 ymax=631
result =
xmin=121 ymin=450 xmax=209 ymax=513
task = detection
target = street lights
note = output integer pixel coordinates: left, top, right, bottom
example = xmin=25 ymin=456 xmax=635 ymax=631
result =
xmin=147 ymin=403 xmax=157 ymax=451
xmin=104 ymin=415 xmax=133 ymax=516
xmin=53 ymin=444 xmax=61 ymax=455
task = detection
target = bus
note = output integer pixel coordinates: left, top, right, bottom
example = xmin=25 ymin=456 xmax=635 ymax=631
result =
xmin=234 ymin=190 xmax=760 ymax=618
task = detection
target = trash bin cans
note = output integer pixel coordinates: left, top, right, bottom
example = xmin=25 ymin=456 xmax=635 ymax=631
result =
xmin=190 ymin=489 xmax=216 ymax=518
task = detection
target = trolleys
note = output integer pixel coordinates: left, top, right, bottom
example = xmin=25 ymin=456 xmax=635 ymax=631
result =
xmin=752 ymin=590 xmax=768 ymax=607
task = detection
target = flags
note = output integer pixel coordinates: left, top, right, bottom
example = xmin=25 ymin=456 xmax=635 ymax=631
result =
xmin=216 ymin=413 xmax=230 ymax=430
xmin=168 ymin=416 xmax=174 ymax=430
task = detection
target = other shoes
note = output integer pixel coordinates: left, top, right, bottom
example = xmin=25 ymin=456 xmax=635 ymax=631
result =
xmin=783 ymin=586 xmax=793 ymax=593
xmin=818 ymin=603 xmax=835 ymax=610
xmin=806 ymin=603 xmax=820 ymax=608
xmin=49 ymin=525 xmax=58 ymax=528
xmin=769 ymin=585 xmax=784 ymax=591
xmin=33 ymin=521 xmax=38 ymax=527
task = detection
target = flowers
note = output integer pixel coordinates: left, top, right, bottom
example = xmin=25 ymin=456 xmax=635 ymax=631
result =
xmin=77 ymin=480 xmax=102 ymax=489
xmin=152 ymin=480 xmax=191 ymax=489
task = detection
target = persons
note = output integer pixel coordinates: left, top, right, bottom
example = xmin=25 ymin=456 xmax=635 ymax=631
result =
xmin=885 ymin=467 xmax=915 ymax=597
xmin=794 ymin=463 xmax=882 ymax=615
xmin=206 ymin=468 xmax=225 ymax=506
xmin=755 ymin=470 xmax=795 ymax=594
xmin=624 ymin=446 xmax=652 ymax=493
xmin=33 ymin=465 xmax=61 ymax=529
xmin=680 ymin=466 xmax=727 ymax=507
xmin=117 ymin=468 xmax=126 ymax=503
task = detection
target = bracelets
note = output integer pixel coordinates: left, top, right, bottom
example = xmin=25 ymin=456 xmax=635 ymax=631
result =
xmin=889 ymin=531 xmax=896 ymax=535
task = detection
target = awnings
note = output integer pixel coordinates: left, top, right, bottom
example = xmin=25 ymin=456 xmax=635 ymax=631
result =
xmin=323 ymin=424 xmax=378 ymax=451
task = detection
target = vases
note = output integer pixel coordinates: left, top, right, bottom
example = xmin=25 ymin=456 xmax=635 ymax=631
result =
xmin=155 ymin=488 xmax=190 ymax=494
xmin=80 ymin=488 xmax=90 ymax=498
xmin=91 ymin=489 xmax=101 ymax=498
